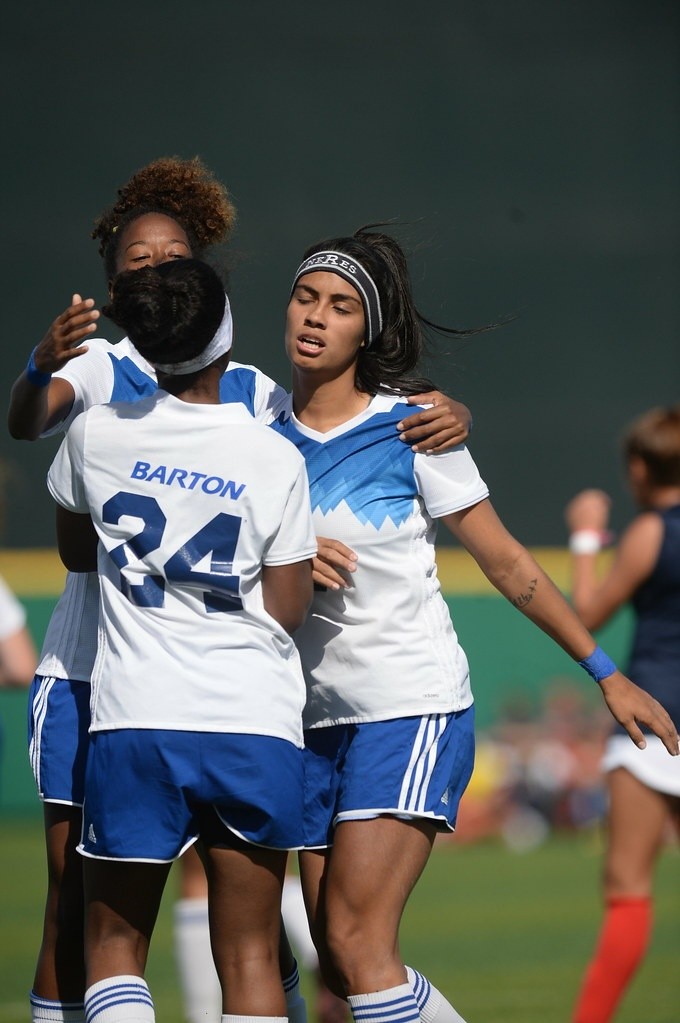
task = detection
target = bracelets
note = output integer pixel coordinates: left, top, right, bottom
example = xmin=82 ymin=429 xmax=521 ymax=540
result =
xmin=577 ymin=646 xmax=618 ymax=681
xmin=572 ymin=531 xmax=599 ymax=551
xmin=27 ymin=346 xmax=51 ymax=386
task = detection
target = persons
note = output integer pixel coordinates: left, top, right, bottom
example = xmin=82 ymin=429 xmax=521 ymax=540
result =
xmin=7 ymin=155 xmax=475 ymax=1023
xmin=0 ymin=576 xmax=36 ymax=689
xmin=45 ymin=257 xmax=317 ymax=1023
xmin=262 ymin=223 xmax=679 ymax=1023
xmin=568 ymin=410 xmax=680 ymax=1022
